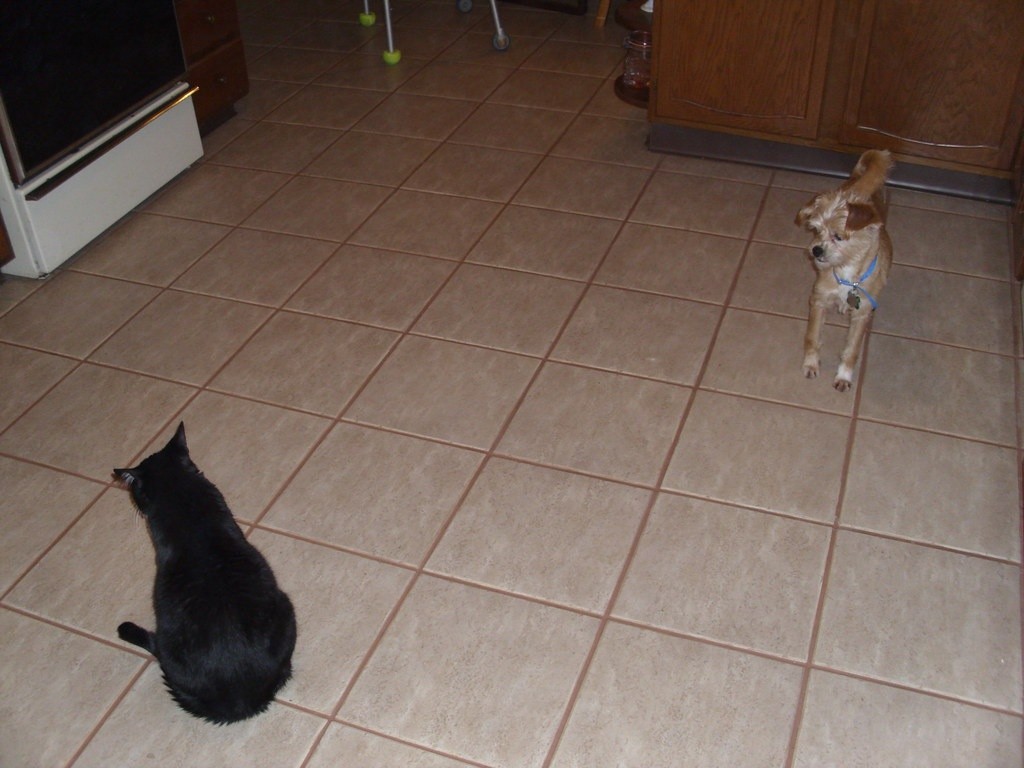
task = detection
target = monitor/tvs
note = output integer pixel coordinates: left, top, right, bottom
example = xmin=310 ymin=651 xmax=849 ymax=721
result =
xmin=0 ymin=0 xmax=188 ymax=185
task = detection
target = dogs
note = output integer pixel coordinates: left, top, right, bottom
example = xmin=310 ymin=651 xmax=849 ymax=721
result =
xmin=794 ymin=150 xmax=894 ymax=392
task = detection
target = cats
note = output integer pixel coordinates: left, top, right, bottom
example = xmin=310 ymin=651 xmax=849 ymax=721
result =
xmin=113 ymin=421 xmax=298 ymax=726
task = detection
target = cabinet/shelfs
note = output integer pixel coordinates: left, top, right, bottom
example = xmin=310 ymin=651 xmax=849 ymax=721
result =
xmin=174 ymin=1 xmax=251 ymax=136
xmin=654 ymin=0 xmax=1024 ymax=178
